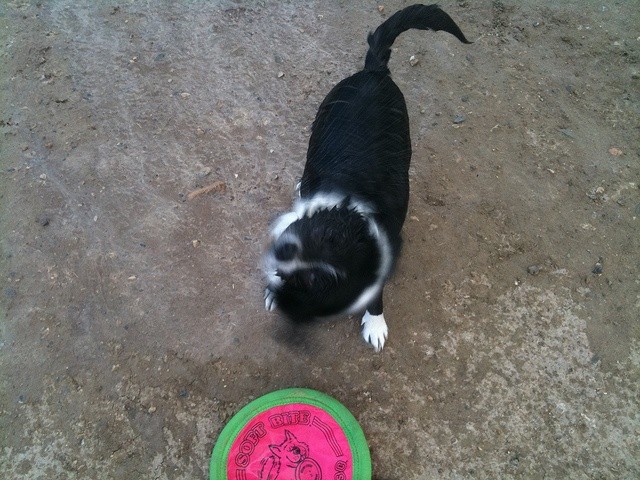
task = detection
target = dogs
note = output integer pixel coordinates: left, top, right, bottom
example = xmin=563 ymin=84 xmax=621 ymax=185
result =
xmin=257 ymin=0 xmax=476 ymax=356
